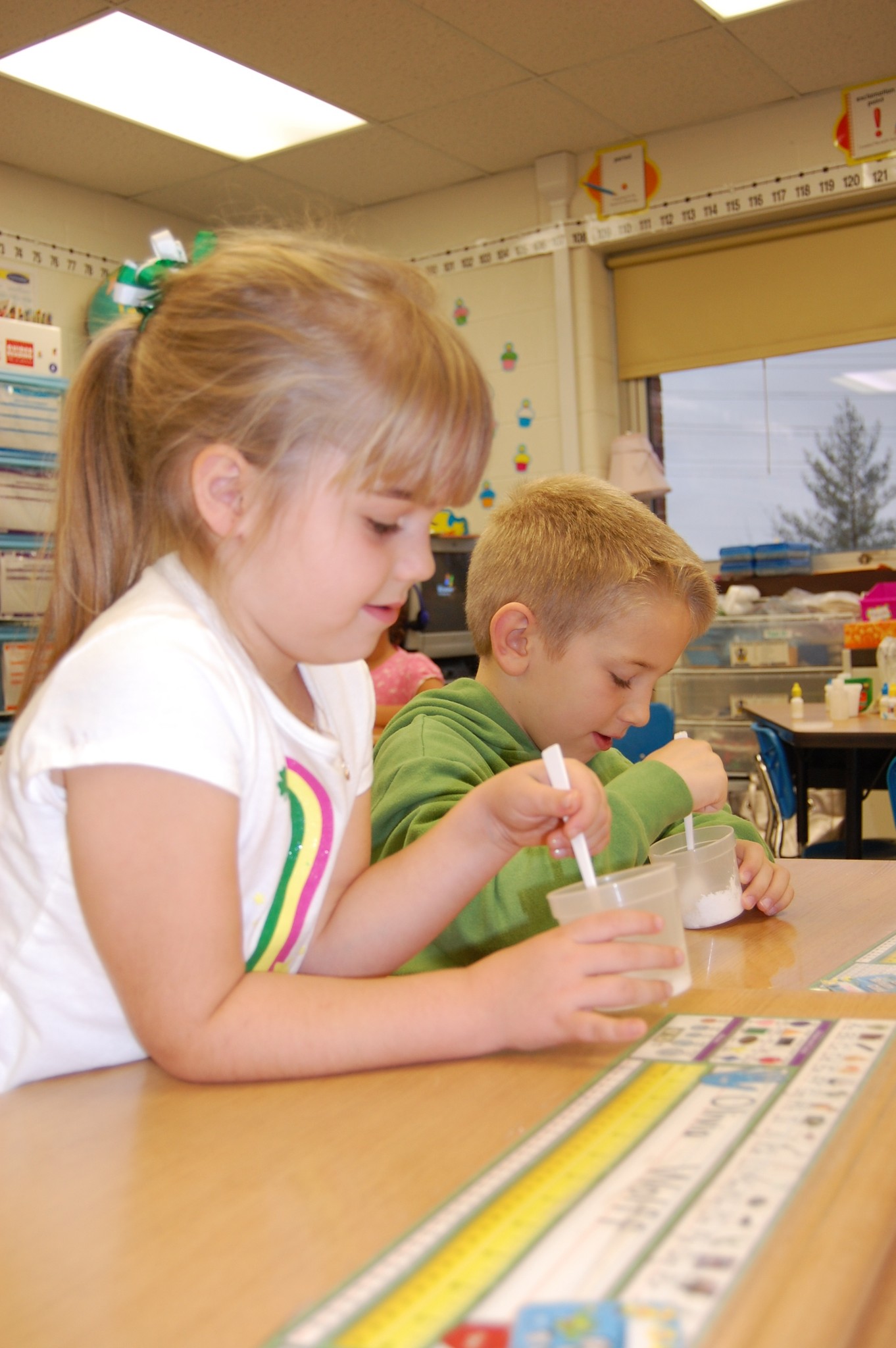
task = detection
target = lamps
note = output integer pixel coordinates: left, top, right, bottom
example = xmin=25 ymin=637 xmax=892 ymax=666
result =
xmin=607 ymin=431 xmax=670 ymax=501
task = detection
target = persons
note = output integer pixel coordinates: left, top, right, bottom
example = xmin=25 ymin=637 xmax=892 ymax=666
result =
xmin=371 ymin=470 xmax=797 ymax=976
xmin=365 ymin=601 xmax=450 ymax=749
xmin=1 ymin=230 xmax=688 ymax=1097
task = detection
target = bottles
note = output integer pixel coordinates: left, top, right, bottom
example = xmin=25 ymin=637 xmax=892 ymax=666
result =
xmin=880 ymin=682 xmax=896 ymax=722
xmin=791 ymin=681 xmax=804 ymax=719
xmin=829 ymin=677 xmax=848 ymax=721
xmin=824 ymin=683 xmax=832 ymax=711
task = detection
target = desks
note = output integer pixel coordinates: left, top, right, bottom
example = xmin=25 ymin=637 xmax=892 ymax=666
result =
xmin=0 ymin=860 xmax=895 ymax=1347
xmin=738 ymin=700 xmax=896 ymax=860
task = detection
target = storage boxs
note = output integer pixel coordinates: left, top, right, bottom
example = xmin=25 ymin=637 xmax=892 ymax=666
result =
xmin=1 ymin=642 xmax=55 ymax=713
xmin=0 ymin=318 xmax=62 ymax=377
xmin=1 ymin=466 xmax=60 ymax=533
xmin=0 ymin=553 xmax=59 ymax=619
xmin=666 ymin=582 xmax=896 ymax=815
xmin=0 ymin=384 xmax=61 ymax=453
xmin=718 ymin=543 xmax=814 ymax=583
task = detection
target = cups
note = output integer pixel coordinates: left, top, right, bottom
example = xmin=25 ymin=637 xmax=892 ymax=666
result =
xmin=648 ymin=825 xmax=745 ymax=929
xmin=545 ymin=862 xmax=692 ymax=1012
xmin=841 ymin=683 xmax=863 ymax=717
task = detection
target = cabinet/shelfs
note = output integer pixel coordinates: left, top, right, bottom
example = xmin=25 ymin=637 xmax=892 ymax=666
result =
xmin=0 ymin=371 xmax=72 ymax=741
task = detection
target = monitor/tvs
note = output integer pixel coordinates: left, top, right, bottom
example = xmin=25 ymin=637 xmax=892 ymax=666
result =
xmin=389 ymin=539 xmax=481 ymax=656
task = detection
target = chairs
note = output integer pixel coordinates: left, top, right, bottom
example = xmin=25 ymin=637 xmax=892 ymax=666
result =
xmin=747 ymin=719 xmax=896 ymax=859
